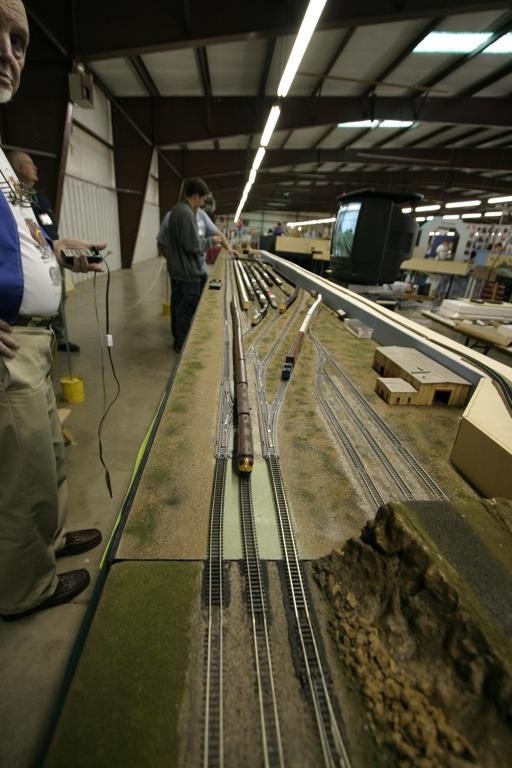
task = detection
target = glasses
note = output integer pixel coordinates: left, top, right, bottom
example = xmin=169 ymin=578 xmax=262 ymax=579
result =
xmin=10 ymin=181 xmax=39 ymax=210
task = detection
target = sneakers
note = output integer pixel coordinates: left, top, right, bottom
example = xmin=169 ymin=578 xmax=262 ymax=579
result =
xmin=57 ymin=342 xmax=80 ymax=353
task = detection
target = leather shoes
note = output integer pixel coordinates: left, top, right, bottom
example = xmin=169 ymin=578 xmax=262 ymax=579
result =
xmin=55 ymin=528 xmax=102 ymax=559
xmin=1 ymin=568 xmax=91 ymax=624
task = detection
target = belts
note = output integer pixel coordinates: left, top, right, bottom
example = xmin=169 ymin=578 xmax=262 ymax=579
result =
xmin=5 ymin=313 xmax=61 ymax=328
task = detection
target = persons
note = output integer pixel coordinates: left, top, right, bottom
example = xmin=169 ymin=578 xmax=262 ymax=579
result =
xmin=167 ymin=177 xmax=220 ymax=350
xmin=272 ymin=222 xmax=285 ymax=239
xmin=156 ymin=197 xmax=240 ymax=286
xmin=432 ymin=240 xmax=454 ymax=261
xmin=0 ymin=0 xmax=102 ymax=620
xmin=7 ymin=149 xmax=81 ymax=352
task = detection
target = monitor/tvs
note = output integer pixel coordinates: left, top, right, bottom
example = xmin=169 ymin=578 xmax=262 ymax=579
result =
xmin=330 ymin=187 xmax=425 ymax=285
